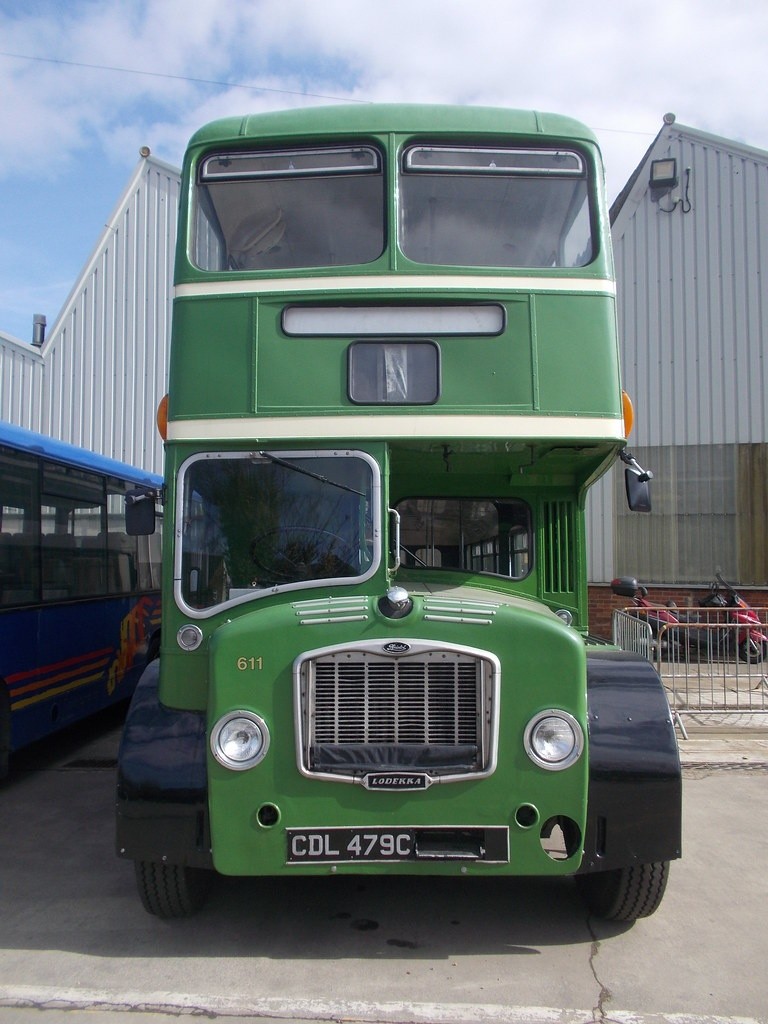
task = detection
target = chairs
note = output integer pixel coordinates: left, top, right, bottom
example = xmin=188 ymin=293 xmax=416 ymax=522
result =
xmin=1 ymin=530 xmax=137 ymax=599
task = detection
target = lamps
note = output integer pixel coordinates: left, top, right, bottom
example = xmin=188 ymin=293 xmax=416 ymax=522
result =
xmin=649 ymin=157 xmax=677 ymax=188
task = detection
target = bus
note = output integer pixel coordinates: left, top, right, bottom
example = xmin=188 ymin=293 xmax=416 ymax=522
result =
xmin=124 ymin=106 xmax=683 ymax=925
xmin=1 ymin=422 xmax=205 ymax=756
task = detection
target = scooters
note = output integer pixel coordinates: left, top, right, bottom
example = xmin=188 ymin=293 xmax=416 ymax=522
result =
xmin=609 ymin=573 xmax=768 ymax=665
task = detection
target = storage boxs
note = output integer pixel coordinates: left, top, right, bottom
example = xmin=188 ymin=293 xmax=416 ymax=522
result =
xmin=611 ymin=577 xmax=638 ymax=597
